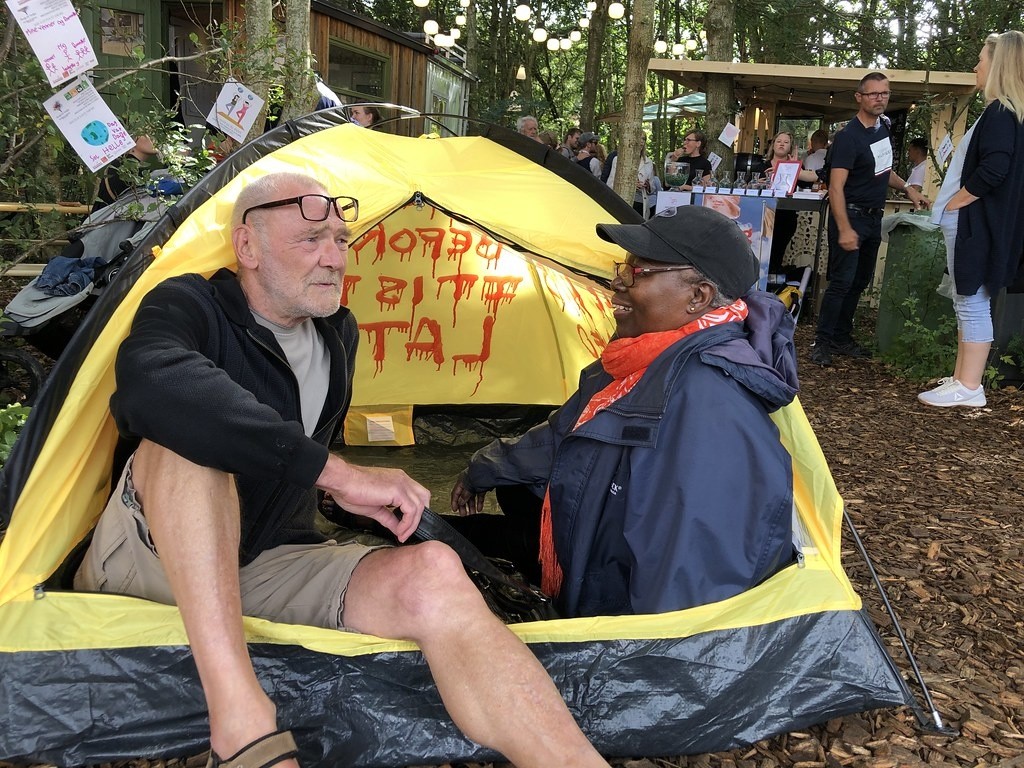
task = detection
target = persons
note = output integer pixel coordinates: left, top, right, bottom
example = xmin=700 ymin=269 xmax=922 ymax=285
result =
xmin=916 ymin=29 xmax=1024 ymax=408
xmin=86 ymin=119 xmax=160 ymax=223
xmin=315 ymin=203 xmax=794 ymax=620
xmin=811 ymin=71 xmax=934 ymax=372
xmin=201 ymin=76 xmax=384 ymax=172
xmin=70 ymin=171 xmax=613 ymax=768
xmin=515 ymin=113 xmax=928 ymax=214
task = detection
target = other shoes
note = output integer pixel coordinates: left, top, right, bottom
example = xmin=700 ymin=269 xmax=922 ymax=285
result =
xmin=768 ymin=274 xmax=776 ymax=284
xmin=809 ymin=342 xmax=873 ymax=368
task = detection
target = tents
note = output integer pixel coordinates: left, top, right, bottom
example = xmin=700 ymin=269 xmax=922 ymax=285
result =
xmin=1 ymin=99 xmax=944 ymax=768
xmin=594 ymin=89 xmax=741 ymax=151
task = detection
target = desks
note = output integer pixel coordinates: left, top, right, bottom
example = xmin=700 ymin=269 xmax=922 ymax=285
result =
xmin=657 ymin=190 xmax=830 ymax=323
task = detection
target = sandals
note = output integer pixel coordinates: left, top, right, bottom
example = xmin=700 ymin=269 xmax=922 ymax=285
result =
xmin=206 ymin=729 xmax=299 ymax=768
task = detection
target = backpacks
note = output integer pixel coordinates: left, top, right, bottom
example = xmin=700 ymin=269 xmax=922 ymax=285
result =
xmin=774 ymin=282 xmax=802 ymax=308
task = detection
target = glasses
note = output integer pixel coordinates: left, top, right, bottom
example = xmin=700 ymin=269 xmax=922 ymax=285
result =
xmin=684 ymin=138 xmax=699 ymax=144
xmin=242 ymin=194 xmax=358 ymax=222
xmin=858 ymin=91 xmax=892 ymax=100
xmin=589 ymin=141 xmax=597 ymax=145
xmin=610 ymin=261 xmax=693 ymax=287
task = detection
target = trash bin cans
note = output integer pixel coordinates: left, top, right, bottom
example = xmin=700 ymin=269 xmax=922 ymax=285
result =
xmin=874 ymin=210 xmax=956 ymax=357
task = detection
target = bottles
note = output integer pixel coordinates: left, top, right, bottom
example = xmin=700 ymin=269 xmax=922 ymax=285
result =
xmin=747 ymin=171 xmax=773 ymax=190
xmin=706 ymin=170 xmax=719 ymax=188
xmin=692 ymin=170 xmax=705 ymax=187
xmin=811 ymin=178 xmax=826 ymax=192
xmin=719 ymin=171 xmax=733 ymax=189
xmin=734 ymin=172 xmax=747 ymax=189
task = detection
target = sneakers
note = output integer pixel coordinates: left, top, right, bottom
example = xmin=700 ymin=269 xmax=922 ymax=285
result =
xmin=918 ymin=377 xmax=986 ymax=407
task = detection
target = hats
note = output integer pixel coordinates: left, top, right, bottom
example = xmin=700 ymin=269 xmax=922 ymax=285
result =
xmin=578 ymin=132 xmax=598 ymax=145
xmin=596 ymin=205 xmax=760 ymax=299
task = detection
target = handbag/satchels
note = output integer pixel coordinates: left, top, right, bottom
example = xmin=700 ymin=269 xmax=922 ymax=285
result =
xmin=394 ymin=502 xmax=558 ymax=623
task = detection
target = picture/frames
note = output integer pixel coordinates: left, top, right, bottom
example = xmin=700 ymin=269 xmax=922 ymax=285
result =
xmin=769 ymin=161 xmax=802 ymax=196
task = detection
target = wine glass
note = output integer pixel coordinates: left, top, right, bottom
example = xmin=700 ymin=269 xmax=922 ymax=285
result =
xmin=666 ymin=162 xmax=690 ymax=192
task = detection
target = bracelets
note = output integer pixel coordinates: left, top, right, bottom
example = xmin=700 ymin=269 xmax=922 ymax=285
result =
xmin=900 ymin=182 xmax=910 ymax=193
